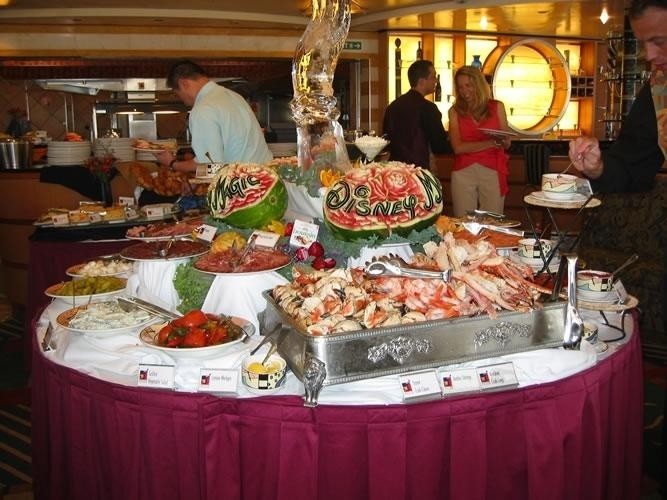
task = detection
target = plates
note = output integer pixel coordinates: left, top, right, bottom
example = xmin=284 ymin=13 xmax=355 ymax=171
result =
xmin=44 ymin=138 xmax=183 ymax=167
xmin=55 ymin=301 xmax=160 ymax=338
xmin=118 ymin=245 xmax=211 ymax=264
xmin=256 ymin=281 xmax=578 ymax=410
xmin=522 ymin=193 xmax=601 ymax=209
xmin=476 ymin=127 xmax=517 ymax=139
xmin=66 ymin=253 xmax=134 ymax=276
xmin=193 ymin=244 xmax=297 ymax=275
xmin=36 ymin=195 xmax=183 ymax=228
xmin=45 ymin=276 xmax=128 ymax=307
xmin=529 ymin=191 xmax=588 ymax=203
xmin=123 ymin=232 xmax=195 ymax=243
xmin=458 ymin=215 xmax=640 ymax=311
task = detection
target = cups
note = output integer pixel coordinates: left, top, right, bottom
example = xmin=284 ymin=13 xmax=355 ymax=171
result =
xmin=542 ymin=172 xmax=581 ymax=200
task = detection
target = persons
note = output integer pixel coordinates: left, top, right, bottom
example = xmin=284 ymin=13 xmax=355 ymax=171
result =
xmin=448 ymin=64 xmax=506 ymax=218
xmin=383 ymin=60 xmax=452 ymax=169
xmin=152 ymin=60 xmax=274 ymax=174
xmin=568 ymin=0 xmax=667 ymax=194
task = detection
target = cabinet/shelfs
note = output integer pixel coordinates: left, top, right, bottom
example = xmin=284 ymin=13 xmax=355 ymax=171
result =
xmin=600 ymin=17 xmax=648 ymax=142
xmin=384 ymin=34 xmax=599 ymax=139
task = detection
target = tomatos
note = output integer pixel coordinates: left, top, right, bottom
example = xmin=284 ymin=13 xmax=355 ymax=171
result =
xmin=158 ymin=310 xmax=228 ymax=347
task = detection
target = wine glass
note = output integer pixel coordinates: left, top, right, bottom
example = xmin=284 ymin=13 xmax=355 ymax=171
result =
xmin=353 ymin=141 xmax=389 ymax=162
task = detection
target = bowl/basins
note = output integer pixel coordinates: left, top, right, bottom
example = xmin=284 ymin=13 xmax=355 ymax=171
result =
xmin=240 ymin=355 xmax=288 ymax=394
xmin=29 ymin=143 xmax=47 ymax=164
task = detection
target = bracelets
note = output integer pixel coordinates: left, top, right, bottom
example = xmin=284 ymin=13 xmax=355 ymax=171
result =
xmin=494 ymin=140 xmax=501 ymax=146
xmin=170 ymin=160 xmax=176 ymax=171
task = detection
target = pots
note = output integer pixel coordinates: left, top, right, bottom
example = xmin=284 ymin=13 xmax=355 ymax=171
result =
xmin=1 ymin=139 xmax=30 ymax=170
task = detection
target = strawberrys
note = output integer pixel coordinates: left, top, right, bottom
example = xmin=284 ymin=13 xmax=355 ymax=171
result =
xmin=285 ymin=224 xmax=294 ymax=235
xmin=296 ymin=242 xmax=336 ymax=270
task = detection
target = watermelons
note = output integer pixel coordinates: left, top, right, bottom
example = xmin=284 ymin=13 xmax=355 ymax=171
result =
xmin=323 ymin=161 xmax=444 ymax=241
xmin=205 ymin=163 xmax=287 ymax=229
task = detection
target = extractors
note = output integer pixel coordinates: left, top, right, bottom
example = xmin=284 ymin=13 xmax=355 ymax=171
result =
xmin=32 ymin=78 xmax=245 ymax=104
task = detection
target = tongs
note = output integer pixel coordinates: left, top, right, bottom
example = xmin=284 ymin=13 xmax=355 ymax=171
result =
xmin=554 ymin=254 xmax=583 ymax=350
xmin=250 ymin=322 xmax=290 ymax=366
xmin=363 ymin=253 xmax=452 ymax=279
xmin=473 ymin=208 xmax=504 ymax=220
xmin=72 ymin=277 xmax=97 ymax=307
xmin=114 ymin=295 xmax=182 ymax=320
